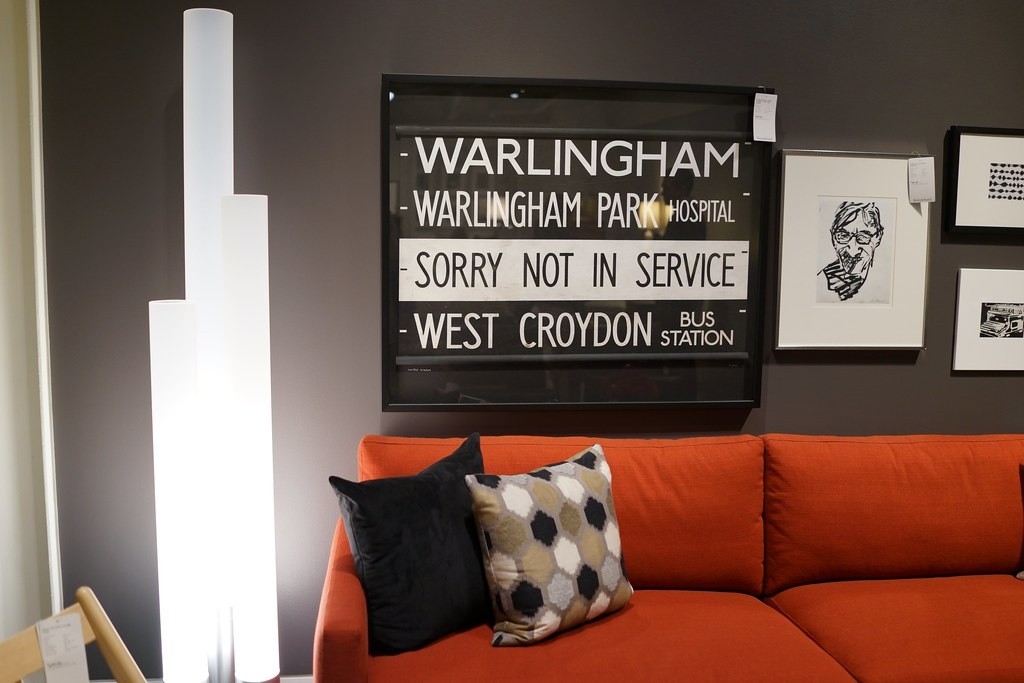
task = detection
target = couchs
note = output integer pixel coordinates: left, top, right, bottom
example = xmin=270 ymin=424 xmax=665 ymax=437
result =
xmin=314 ymin=433 xmax=1024 ymax=683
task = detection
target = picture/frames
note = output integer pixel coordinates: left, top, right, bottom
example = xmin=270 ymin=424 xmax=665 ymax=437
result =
xmin=775 ymin=147 xmax=937 ymax=351
xmin=379 ymin=72 xmax=776 ymax=413
xmin=951 ymin=268 xmax=1024 ymax=371
xmin=941 ymin=125 xmax=1024 ymax=246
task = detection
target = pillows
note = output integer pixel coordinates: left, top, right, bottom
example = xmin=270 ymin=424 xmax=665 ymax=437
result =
xmin=462 ymin=443 xmax=635 ymax=648
xmin=328 ymin=432 xmax=494 ymax=656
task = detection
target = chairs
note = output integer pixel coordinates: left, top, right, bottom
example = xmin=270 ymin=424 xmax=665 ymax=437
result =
xmin=0 ymin=585 xmax=149 ymax=683
